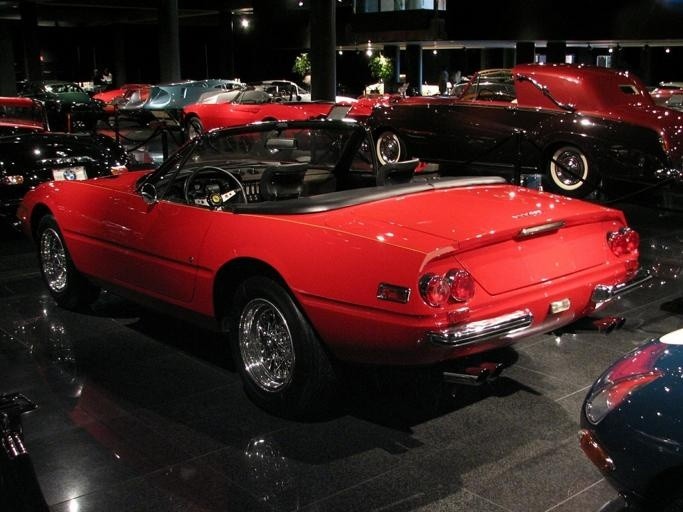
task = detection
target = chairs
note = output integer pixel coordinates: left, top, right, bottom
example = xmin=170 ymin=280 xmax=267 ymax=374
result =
xmin=259 ymin=160 xmax=311 ymax=202
xmin=374 ymin=157 xmax=421 ymax=187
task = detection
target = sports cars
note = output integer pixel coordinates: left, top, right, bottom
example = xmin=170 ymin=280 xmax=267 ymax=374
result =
xmin=344 ymin=67 xmax=683 ymax=198
xmin=17 ymin=116 xmax=645 ymax=413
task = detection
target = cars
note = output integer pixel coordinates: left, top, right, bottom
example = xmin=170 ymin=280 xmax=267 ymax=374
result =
xmin=576 ymin=326 xmax=682 ymax=512
xmin=183 ymin=89 xmax=352 ymax=131
xmin=1 ymin=71 xmax=359 ymax=157
xmin=363 ymin=75 xmax=452 ymax=98
